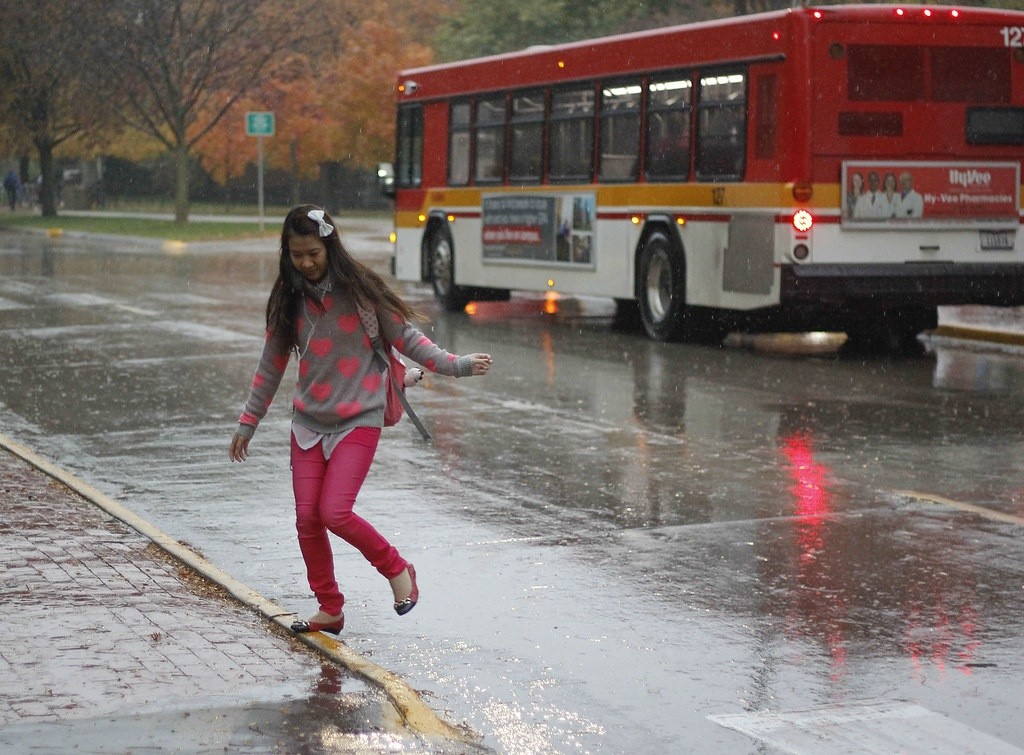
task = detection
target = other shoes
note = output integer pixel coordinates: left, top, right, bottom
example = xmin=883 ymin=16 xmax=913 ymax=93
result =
xmin=291 ymin=610 xmax=344 ymax=634
xmin=393 ymin=564 xmax=418 ymax=615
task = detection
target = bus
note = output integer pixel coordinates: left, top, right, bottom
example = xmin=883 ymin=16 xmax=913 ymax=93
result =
xmin=377 ymin=3 xmax=1024 ymax=348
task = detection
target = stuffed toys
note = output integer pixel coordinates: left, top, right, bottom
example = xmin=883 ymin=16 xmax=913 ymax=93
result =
xmin=403 ymin=366 xmax=425 ymax=387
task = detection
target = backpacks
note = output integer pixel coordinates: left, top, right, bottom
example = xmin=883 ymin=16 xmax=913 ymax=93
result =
xmin=354 ymin=289 xmax=406 ymax=426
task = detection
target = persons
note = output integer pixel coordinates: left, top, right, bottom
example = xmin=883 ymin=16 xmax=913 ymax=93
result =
xmin=215 ymin=203 xmax=492 ymax=636
xmin=664 ymin=130 xmax=690 ymax=174
xmin=847 ymin=171 xmax=923 ymax=219
xmin=2 ymin=169 xmax=20 ymax=211
